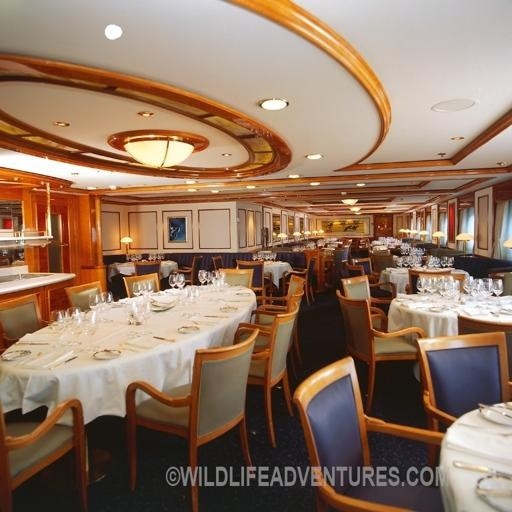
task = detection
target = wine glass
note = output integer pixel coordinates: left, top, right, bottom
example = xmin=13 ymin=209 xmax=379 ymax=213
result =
xmin=50 ymin=308 xmax=98 ymax=354
xmin=184 ymin=284 xmax=199 ymax=305
xmin=417 ymin=273 xmax=504 ymax=307
xmin=127 ymin=300 xmax=153 ymax=336
xmin=199 ymin=268 xmax=227 ymax=295
xmin=168 ymin=273 xmax=185 ymax=298
xmin=394 ymin=255 xmax=455 ymax=271
xmin=131 ymin=279 xmax=154 ymax=300
xmin=90 ymin=291 xmax=112 ymax=323
xmin=126 ymin=252 xmax=165 ymax=263
xmin=252 ymin=252 xmax=277 ymax=264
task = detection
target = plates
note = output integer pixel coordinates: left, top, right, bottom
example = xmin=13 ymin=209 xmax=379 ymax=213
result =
xmin=2 ymin=348 xmax=33 ymax=363
xmin=177 ymin=325 xmax=202 ymax=335
xmin=236 ymin=293 xmax=251 ymax=297
xmin=219 ymin=306 xmax=239 ymax=314
xmin=480 ymin=403 xmax=512 ymax=427
xmin=93 ymin=350 xmax=122 ymax=360
xmin=475 ymin=473 xmax=512 ymax=511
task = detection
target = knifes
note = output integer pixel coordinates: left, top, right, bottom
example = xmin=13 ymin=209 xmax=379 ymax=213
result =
xmin=204 ymin=314 xmax=229 ymax=319
xmin=50 ymin=355 xmax=79 ymax=369
xmin=154 ymin=336 xmax=175 ymax=342
xmin=478 ymin=401 xmax=511 ymax=419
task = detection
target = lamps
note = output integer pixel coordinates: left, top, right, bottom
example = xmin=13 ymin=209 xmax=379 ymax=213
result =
xmin=122 ymin=135 xmax=195 ymax=169
xmin=278 ymin=229 xmax=325 ymax=248
xmin=399 ymin=226 xmax=473 ymax=258
xmin=341 ymin=199 xmax=363 ymax=215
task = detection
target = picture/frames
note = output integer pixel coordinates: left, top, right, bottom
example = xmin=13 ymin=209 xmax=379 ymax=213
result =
xmin=167 ymin=216 xmax=187 ymax=242
